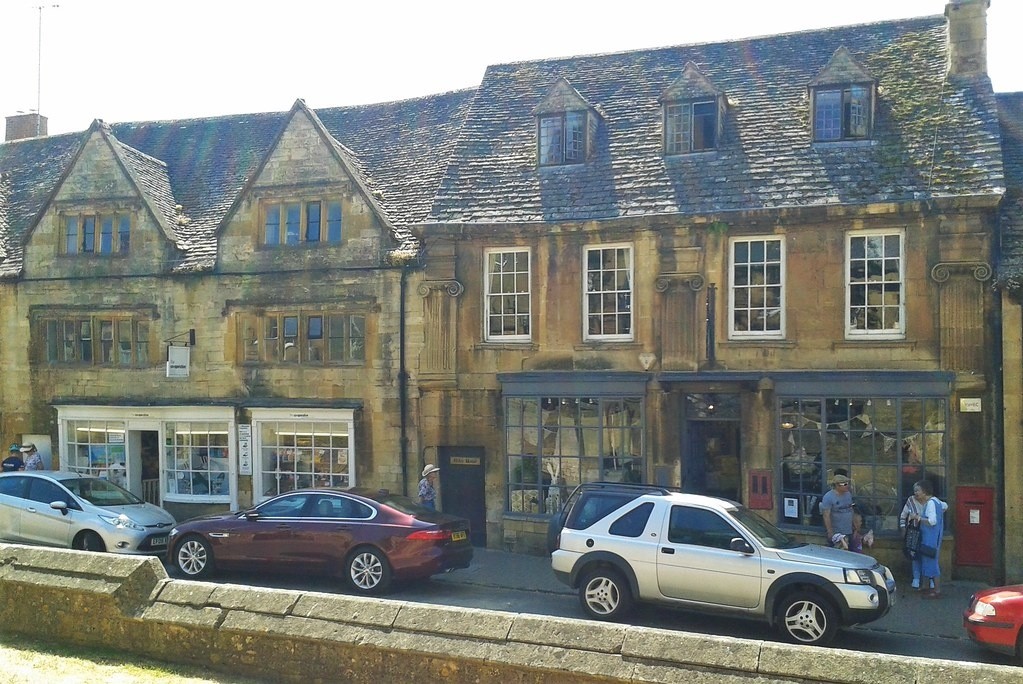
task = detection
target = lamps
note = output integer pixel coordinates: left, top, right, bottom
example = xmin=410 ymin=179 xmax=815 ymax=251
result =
xmin=886 ymin=399 xmax=892 ymax=405
xmin=835 ymin=399 xmax=840 ymax=406
xmin=866 ymin=399 xmax=871 ymax=406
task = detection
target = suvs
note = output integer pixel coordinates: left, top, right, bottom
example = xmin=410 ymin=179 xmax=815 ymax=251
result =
xmin=547 ymin=480 xmax=898 ymax=645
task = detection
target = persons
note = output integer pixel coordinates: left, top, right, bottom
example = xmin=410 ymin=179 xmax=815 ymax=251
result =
xmin=20 ymin=442 xmax=44 ymax=471
xmin=0 ymin=443 xmax=24 ymax=473
xmin=417 ymin=464 xmax=441 ymax=509
xmin=822 ymin=474 xmax=948 ymax=598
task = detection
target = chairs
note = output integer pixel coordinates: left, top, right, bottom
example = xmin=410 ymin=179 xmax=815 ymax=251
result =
xmin=319 ymin=501 xmax=334 ymax=517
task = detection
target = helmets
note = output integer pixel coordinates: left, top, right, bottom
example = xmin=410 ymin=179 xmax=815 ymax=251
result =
xmin=9 ymin=443 xmax=21 ymax=452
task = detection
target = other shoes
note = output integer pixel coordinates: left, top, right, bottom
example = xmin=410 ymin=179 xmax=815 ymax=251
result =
xmin=912 ymin=579 xmax=920 ymax=587
xmin=929 ymin=579 xmax=934 ymax=588
xmin=922 ymin=590 xmax=940 ymax=598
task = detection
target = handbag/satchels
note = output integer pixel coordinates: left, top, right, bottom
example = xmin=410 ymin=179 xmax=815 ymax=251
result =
xmin=904 ymin=520 xmax=921 ymax=550
xmin=918 ymin=543 xmax=937 ymax=557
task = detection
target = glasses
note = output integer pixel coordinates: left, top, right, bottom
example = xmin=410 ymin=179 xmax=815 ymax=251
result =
xmin=839 ymin=483 xmax=848 ymax=486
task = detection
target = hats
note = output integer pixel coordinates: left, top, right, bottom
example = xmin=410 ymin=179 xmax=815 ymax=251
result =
xmin=19 ymin=442 xmax=37 ymax=453
xmin=832 ymin=475 xmax=849 ymax=483
xmin=422 ymin=464 xmax=440 ymax=476
xmin=832 ymin=533 xmax=845 ymax=544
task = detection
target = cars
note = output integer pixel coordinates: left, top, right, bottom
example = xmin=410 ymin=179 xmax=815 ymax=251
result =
xmin=165 ymin=486 xmax=476 ymax=596
xmin=0 ymin=471 xmax=180 ymax=558
xmin=961 ymin=581 xmax=1023 ymax=664
